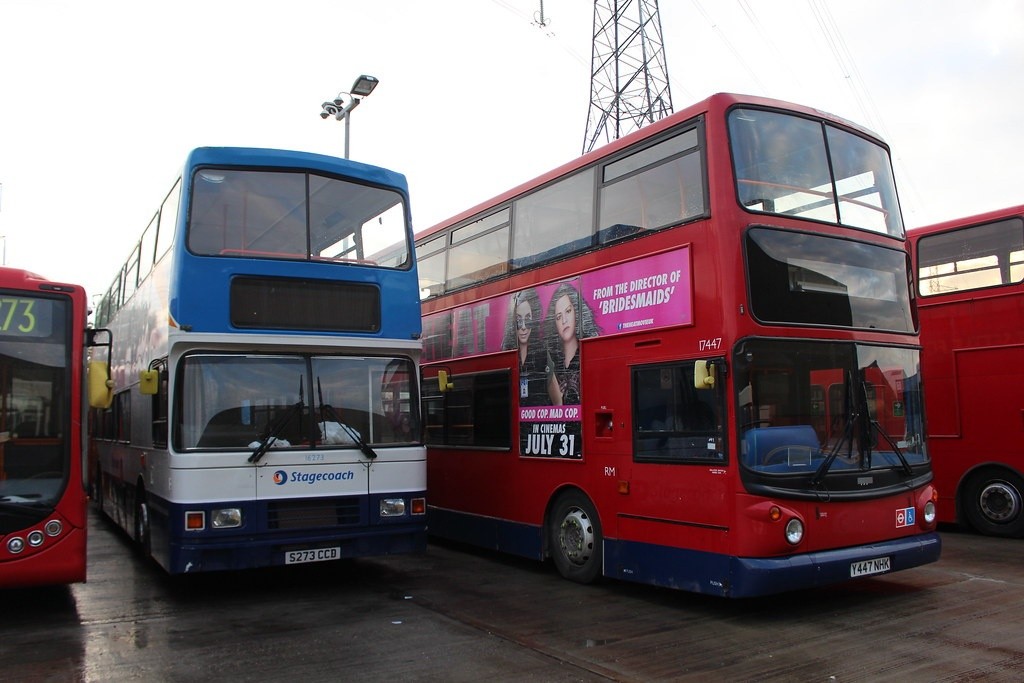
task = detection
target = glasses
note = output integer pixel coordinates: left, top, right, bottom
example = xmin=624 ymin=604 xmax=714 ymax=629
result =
xmin=516 ymin=318 xmax=536 ymax=330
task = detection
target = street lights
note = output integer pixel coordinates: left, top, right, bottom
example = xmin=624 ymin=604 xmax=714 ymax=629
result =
xmin=321 ymin=75 xmax=379 ymax=263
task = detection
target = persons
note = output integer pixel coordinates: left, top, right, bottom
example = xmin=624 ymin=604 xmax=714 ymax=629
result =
xmin=500 ymin=290 xmax=563 ymax=456
xmin=539 ymin=283 xmax=607 ymax=459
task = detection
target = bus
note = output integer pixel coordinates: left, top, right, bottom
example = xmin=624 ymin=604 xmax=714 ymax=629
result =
xmin=810 ymin=204 xmax=1024 ymax=539
xmin=0 ymin=266 xmax=115 ymax=593
xmin=376 ymin=92 xmax=940 ymax=600
xmin=87 ymin=144 xmax=454 ymax=574
